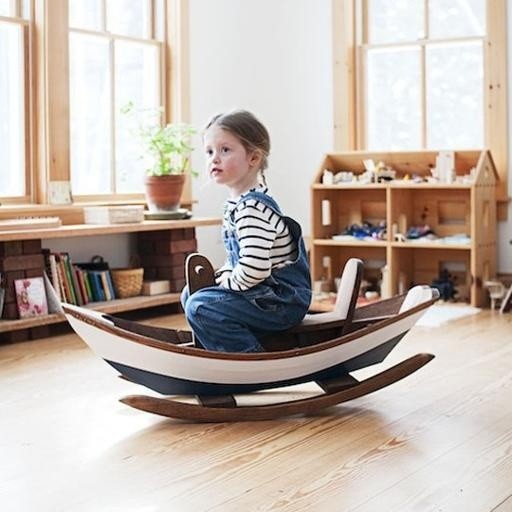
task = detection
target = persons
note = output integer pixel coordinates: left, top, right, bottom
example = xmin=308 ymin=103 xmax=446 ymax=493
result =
xmin=178 ymin=107 xmax=314 ymax=356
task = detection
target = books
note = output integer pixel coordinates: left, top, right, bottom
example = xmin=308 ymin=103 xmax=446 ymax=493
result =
xmin=12 ymin=250 xmax=119 ymax=320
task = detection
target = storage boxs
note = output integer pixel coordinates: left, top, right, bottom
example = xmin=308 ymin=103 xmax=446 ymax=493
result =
xmin=313 ymin=149 xmax=501 ymax=307
xmin=83 ymin=205 xmax=143 ymax=224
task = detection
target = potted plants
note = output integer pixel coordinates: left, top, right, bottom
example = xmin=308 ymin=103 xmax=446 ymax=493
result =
xmin=121 ymin=102 xmax=200 ymax=211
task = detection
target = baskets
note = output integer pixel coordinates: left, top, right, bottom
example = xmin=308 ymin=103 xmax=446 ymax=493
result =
xmin=110 ymin=254 xmax=144 ymax=298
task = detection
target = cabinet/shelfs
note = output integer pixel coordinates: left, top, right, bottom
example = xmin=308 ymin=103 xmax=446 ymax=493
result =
xmin=0 ymin=216 xmax=222 ymax=349
